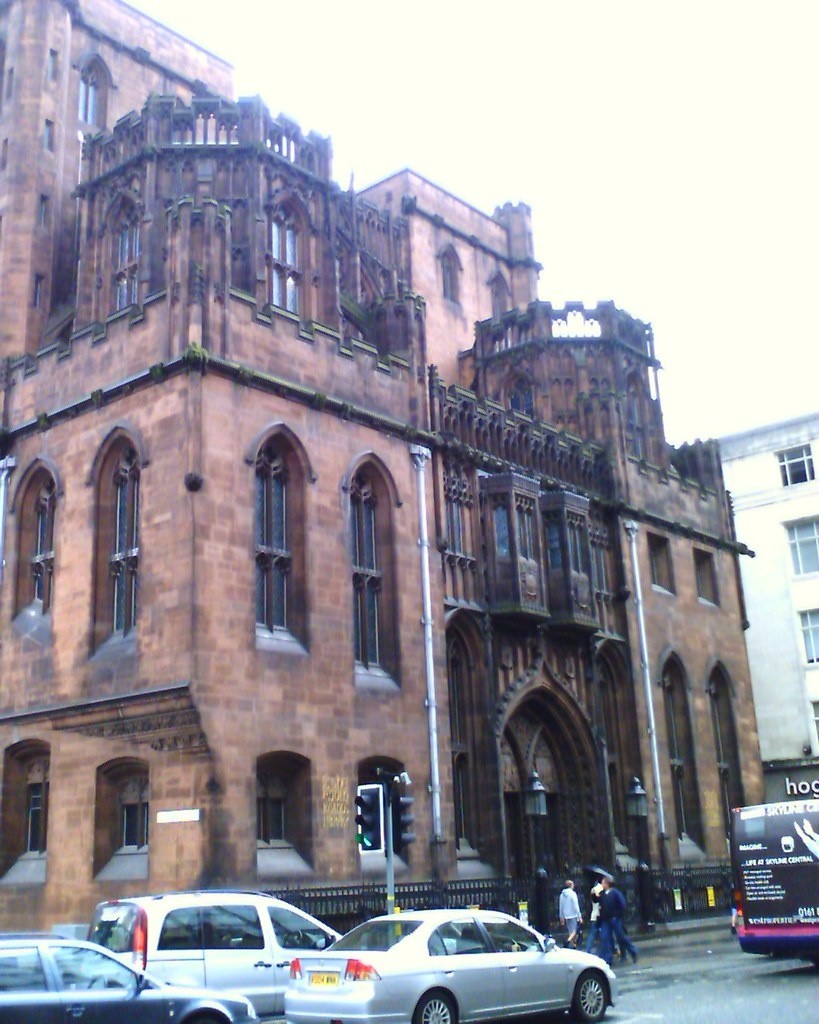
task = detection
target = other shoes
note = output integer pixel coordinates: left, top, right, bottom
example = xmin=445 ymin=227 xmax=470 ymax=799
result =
xmin=730 ymin=926 xmax=737 ymax=935
xmin=633 ymin=954 xmax=637 ymax=963
xmin=613 ymin=951 xmax=620 ymax=957
xmin=563 ymin=941 xmax=569 ymax=948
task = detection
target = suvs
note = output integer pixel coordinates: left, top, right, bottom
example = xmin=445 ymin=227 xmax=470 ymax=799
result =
xmin=0 ymin=932 xmax=261 ymax=1024
xmin=86 ymin=889 xmax=344 ymax=1014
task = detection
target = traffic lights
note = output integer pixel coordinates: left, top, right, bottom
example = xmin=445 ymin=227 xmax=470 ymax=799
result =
xmin=391 ymin=792 xmax=417 ymax=855
xmin=354 ymin=787 xmax=381 ymax=851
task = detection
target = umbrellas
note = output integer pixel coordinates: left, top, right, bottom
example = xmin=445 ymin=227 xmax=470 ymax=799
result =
xmin=582 ymin=865 xmax=616 ymax=885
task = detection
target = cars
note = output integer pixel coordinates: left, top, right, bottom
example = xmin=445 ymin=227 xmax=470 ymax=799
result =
xmin=284 ymin=908 xmax=617 ymax=1024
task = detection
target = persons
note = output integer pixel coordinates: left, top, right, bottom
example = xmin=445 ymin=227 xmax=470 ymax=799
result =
xmin=730 ymin=883 xmax=737 ymax=935
xmin=559 ymin=880 xmax=583 ymax=949
xmin=587 ymin=877 xmax=638 ymax=965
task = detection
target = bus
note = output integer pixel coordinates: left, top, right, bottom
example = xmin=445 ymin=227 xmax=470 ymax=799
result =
xmin=728 ymin=799 xmax=818 ymax=964
